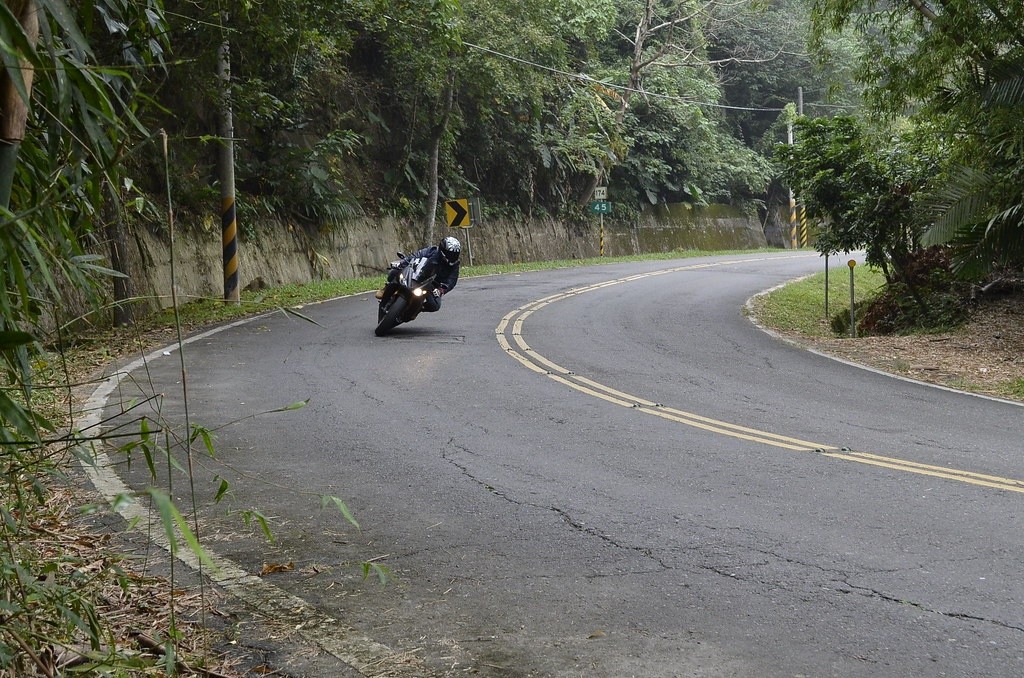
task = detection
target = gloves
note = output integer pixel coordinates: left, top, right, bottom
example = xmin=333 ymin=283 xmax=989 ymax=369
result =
xmin=431 ymin=288 xmax=441 ymax=298
xmin=391 ymin=260 xmax=401 ymax=269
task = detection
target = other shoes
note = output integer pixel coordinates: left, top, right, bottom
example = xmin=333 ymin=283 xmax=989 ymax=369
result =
xmin=375 ymin=288 xmax=386 ymax=298
xmin=411 ymin=306 xmax=422 ymax=319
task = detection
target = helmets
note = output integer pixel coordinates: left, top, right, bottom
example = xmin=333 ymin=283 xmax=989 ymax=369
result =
xmin=439 ymin=236 xmax=461 ymax=267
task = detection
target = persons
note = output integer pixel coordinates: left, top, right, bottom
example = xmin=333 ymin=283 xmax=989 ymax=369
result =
xmin=375 ymin=236 xmax=461 ymax=312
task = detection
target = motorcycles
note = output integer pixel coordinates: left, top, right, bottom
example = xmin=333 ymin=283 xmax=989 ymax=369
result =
xmin=374 ymin=251 xmax=446 ymax=338
